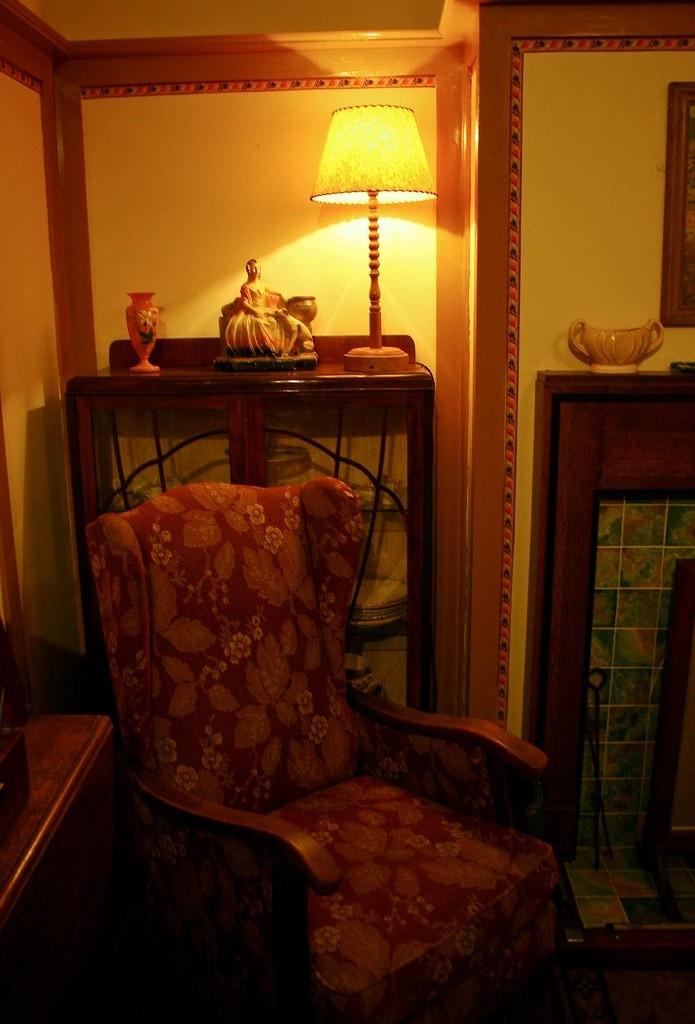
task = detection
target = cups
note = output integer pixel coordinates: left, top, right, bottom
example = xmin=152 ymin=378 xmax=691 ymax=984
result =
xmin=144 ymin=487 xmax=162 ymax=498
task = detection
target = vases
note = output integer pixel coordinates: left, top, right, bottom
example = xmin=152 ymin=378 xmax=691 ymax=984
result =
xmin=125 ymin=292 xmax=160 ymax=373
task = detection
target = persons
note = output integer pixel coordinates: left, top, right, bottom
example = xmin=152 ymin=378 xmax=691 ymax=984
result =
xmin=225 ymin=257 xmax=316 ymax=359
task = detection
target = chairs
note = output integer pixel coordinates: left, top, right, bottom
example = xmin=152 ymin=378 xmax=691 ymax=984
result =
xmin=85 ymin=477 xmax=561 ymax=1024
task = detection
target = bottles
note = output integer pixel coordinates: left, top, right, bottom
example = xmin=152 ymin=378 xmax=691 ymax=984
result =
xmin=381 ymin=475 xmax=394 ymax=505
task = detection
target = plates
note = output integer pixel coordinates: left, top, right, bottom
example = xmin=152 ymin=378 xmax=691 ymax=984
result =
xmin=349 ymin=577 xmax=407 ymax=627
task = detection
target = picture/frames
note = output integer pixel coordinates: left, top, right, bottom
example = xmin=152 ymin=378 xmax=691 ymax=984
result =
xmin=659 ymin=82 xmax=695 ymax=328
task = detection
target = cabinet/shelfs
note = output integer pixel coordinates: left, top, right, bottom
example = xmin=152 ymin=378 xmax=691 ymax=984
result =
xmin=65 ymin=334 xmax=436 ymax=711
xmin=0 ymin=712 xmax=117 ymax=1024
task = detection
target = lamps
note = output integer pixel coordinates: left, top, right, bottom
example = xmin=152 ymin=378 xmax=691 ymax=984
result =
xmin=311 ymin=103 xmax=439 ymax=373
